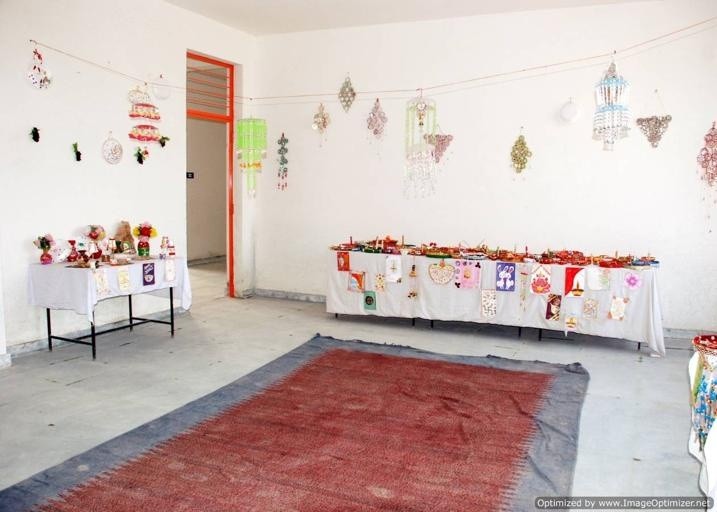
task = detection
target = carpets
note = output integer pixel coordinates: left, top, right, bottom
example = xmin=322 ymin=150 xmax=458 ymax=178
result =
xmin=7 ymin=334 xmax=590 ymax=510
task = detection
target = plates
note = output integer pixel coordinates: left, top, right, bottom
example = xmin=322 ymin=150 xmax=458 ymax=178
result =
xmin=461 ymin=252 xmax=488 ymax=260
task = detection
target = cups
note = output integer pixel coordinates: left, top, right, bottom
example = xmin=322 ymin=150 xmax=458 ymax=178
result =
xmin=399 ymin=248 xmax=410 ymax=256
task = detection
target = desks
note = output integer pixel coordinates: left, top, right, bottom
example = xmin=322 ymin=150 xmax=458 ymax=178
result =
xmin=326 ymin=241 xmax=658 ymax=350
xmin=26 ymin=257 xmax=181 ymax=360
